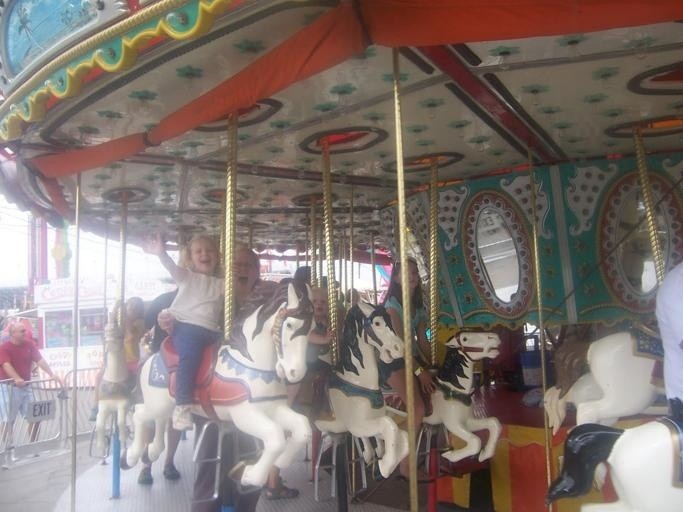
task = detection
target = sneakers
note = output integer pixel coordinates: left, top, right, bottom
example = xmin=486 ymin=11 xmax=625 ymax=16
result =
xmin=136 ymin=466 xmax=154 ymax=484
xmin=162 ymin=463 xmax=180 ymax=480
xmin=170 ymin=405 xmax=194 ymax=431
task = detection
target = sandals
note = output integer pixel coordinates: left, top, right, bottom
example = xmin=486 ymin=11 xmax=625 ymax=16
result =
xmin=265 ymin=483 xmax=300 ymax=501
xmin=397 ymin=470 xmax=435 ymax=486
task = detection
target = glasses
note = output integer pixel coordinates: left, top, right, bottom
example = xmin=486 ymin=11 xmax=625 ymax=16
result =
xmin=394 ymin=256 xmax=417 ymax=266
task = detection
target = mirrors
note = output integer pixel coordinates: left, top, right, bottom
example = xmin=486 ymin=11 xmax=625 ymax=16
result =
xmin=594 ymin=167 xmax=682 ymax=315
xmin=462 ymin=189 xmax=534 ymax=320
